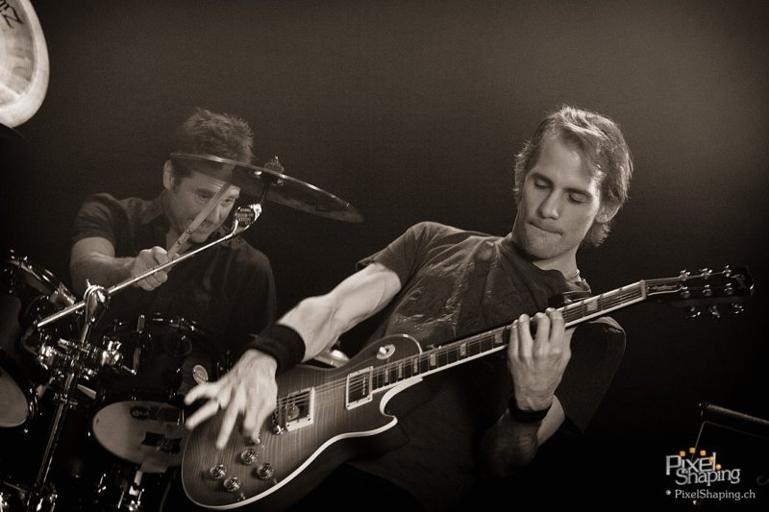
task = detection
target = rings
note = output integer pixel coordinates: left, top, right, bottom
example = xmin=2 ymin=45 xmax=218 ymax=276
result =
xmin=153 ymin=272 xmax=158 ymax=278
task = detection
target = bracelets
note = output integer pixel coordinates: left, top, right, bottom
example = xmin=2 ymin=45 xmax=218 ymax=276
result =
xmin=240 ymin=322 xmax=306 ymax=371
xmin=507 ymin=393 xmax=554 ymax=424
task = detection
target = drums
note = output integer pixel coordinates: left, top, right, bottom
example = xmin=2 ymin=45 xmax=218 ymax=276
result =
xmin=0 ymin=250 xmax=82 ymax=428
xmin=90 ymin=312 xmax=231 ymax=467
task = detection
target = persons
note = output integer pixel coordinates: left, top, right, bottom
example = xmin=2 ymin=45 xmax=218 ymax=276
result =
xmin=182 ymin=102 xmax=635 ymax=511
xmin=67 ymin=108 xmax=278 ymax=398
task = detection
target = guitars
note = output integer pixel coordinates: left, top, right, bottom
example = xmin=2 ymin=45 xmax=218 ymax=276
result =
xmin=180 ymin=265 xmax=749 ymax=510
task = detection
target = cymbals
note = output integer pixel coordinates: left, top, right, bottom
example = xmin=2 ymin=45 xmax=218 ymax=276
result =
xmin=0 ymin=0 xmax=49 ymax=129
xmin=171 ymin=153 xmax=366 ymax=225
